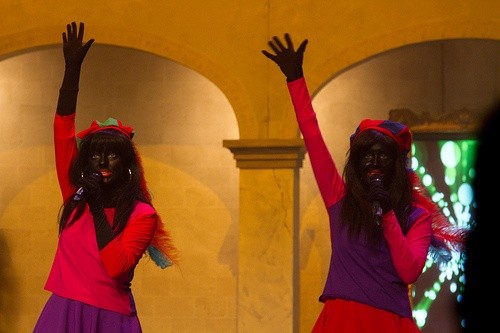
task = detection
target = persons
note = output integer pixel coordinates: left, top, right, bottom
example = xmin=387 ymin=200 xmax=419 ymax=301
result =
xmin=32 ymin=20 xmax=181 ymax=333
xmin=422 ymin=104 xmax=500 ymax=333
xmin=260 ymin=32 xmax=466 ymax=333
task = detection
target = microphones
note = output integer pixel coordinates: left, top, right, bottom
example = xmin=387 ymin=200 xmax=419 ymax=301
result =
xmin=71 ymin=170 xmax=102 ymax=202
xmin=370 ymin=174 xmax=383 ymax=226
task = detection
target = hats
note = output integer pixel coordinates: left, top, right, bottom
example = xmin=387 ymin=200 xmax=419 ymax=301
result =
xmin=349 ymin=118 xmax=412 ymax=153
xmin=75 ymin=117 xmax=135 ymax=152
